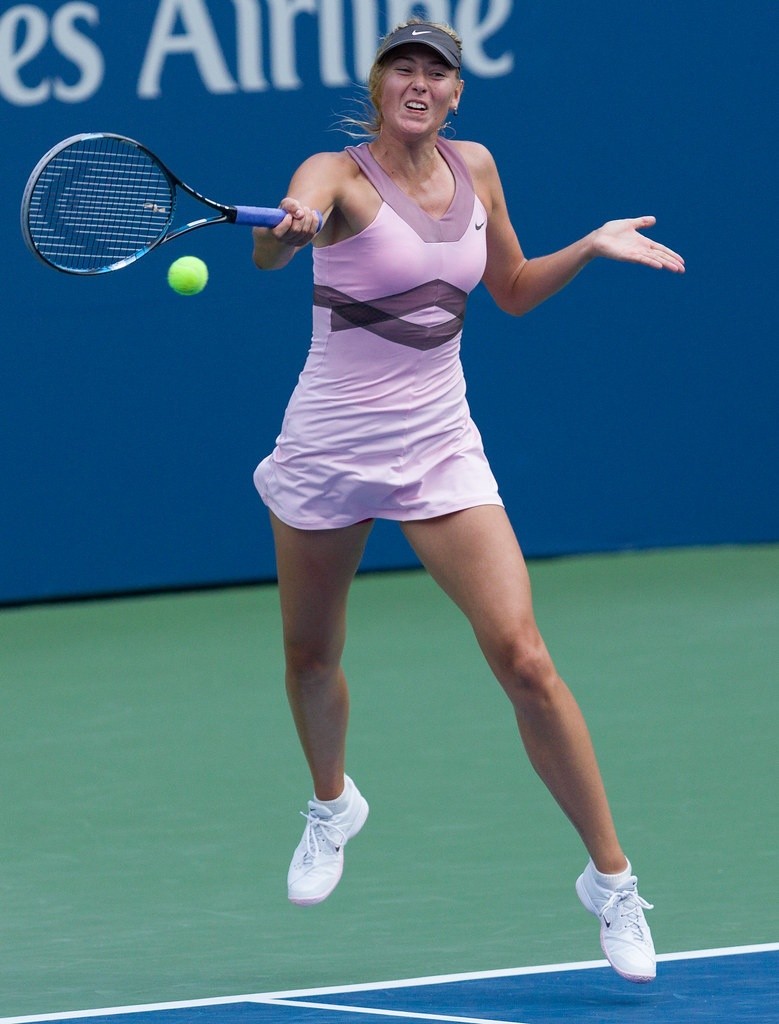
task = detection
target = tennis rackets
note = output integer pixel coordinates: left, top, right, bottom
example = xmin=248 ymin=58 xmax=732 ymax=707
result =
xmin=19 ymin=130 xmax=326 ymax=277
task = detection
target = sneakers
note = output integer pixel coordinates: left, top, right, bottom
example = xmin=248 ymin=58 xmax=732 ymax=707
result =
xmin=574 ymin=863 xmax=658 ymax=982
xmin=286 ymin=772 xmax=370 ymax=903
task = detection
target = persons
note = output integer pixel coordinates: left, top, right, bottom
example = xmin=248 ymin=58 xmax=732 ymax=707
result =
xmin=253 ymin=20 xmax=686 ymax=983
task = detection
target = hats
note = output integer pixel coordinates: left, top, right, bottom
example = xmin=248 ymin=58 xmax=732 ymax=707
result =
xmin=374 ymin=25 xmax=461 ymax=70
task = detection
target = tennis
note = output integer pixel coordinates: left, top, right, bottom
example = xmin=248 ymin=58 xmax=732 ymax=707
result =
xmin=167 ymin=255 xmax=210 ymax=297
